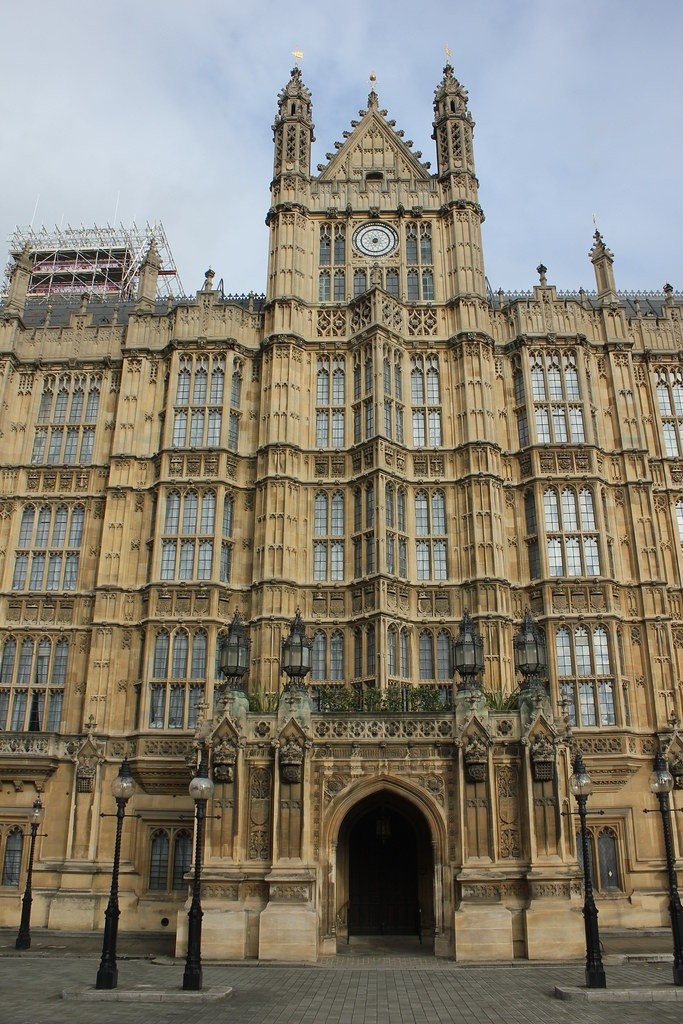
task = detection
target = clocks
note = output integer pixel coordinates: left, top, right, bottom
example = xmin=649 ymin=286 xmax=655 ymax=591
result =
xmin=351 ymin=221 xmax=400 ymax=259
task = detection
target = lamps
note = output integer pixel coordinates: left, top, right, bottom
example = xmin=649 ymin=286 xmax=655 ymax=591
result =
xmin=512 ymin=605 xmax=548 ymax=690
xmin=452 ymin=608 xmax=486 ymax=691
xmin=217 ymin=604 xmax=254 ymax=689
xmin=281 ymin=605 xmax=313 ymax=691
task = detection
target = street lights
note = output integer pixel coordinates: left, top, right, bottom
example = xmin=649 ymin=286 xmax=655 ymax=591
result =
xmin=93 ymin=755 xmax=141 ymax=991
xmin=13 ymin=795 xmax=48 ymax=950
xmin=564 ymin=752 xmax=609 ymax=988
xmin=647 ymin=748 xmax=683 ymax=987
xmin=181 ymin=760 xmax=215 ymax=990
